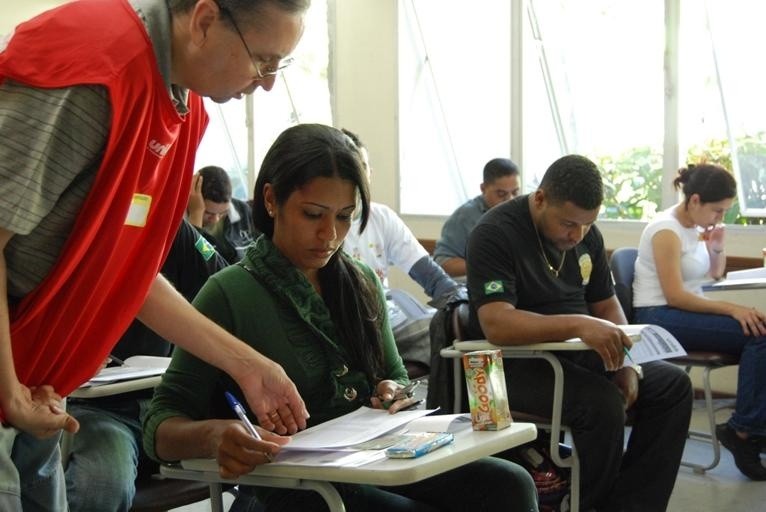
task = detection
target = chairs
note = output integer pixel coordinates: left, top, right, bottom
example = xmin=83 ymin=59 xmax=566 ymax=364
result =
xmin=716 ymin=421 xmax=766 ymax=481
xmin=57 ymin=365 xmax=533 ymax=510
xmin=440 ymin=247 xmax=766 ymax=475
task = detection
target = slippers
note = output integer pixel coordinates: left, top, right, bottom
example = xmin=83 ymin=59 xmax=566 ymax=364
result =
xmin=384 ymin=288 xmax=438 ymax=334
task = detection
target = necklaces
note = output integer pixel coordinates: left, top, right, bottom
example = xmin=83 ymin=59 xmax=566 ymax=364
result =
xmin=533 ymin=218 xmax=566 ymax=277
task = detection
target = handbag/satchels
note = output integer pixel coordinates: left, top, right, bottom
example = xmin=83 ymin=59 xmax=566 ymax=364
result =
xmin=710 ymin=248 xmax=725 ymax=255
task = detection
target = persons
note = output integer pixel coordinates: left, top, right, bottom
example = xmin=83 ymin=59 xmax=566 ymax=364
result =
xmin=465 ymin=155 xmax=693 ymax=512
xmin=433 ymin=159 xmax=520 ymax=277
xmin=1 ymin=0 xmax=311 ymax=511
xmin=182 ymin=165 xmax=259 ymax=267
xmin=340 ymin=129 xmax=458 ymax=311
xmin=61 ymin=218 xmax=229 ymax=510
xmin=142 ymin=123 xmax=540 ymax=512
xmin=632 ymin=164 xmax=766 ymax=480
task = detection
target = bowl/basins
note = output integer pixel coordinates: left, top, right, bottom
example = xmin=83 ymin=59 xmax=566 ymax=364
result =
xmin=533 ymin=218 xmax=566 ymax=277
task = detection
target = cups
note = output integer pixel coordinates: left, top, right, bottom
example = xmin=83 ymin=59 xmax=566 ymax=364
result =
xmin=215 ymin=4 xmax=296 ymax=78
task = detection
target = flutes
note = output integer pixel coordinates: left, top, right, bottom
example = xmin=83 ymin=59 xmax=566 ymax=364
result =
xmin=385 ymin=431 xmax=454 ymax=459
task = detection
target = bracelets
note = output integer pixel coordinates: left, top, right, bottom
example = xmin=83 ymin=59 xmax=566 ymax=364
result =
xmin=710 ymin=248 xmax=725 ymax=255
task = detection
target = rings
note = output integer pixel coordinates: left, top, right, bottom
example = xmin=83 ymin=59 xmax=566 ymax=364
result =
xmin=272 ymin=413 xmax=277 ymax=418
xmin=223 ymin=389 xmax=275 ymax=462
xmin=623 ymin=345 xmax=635 ymax=364
